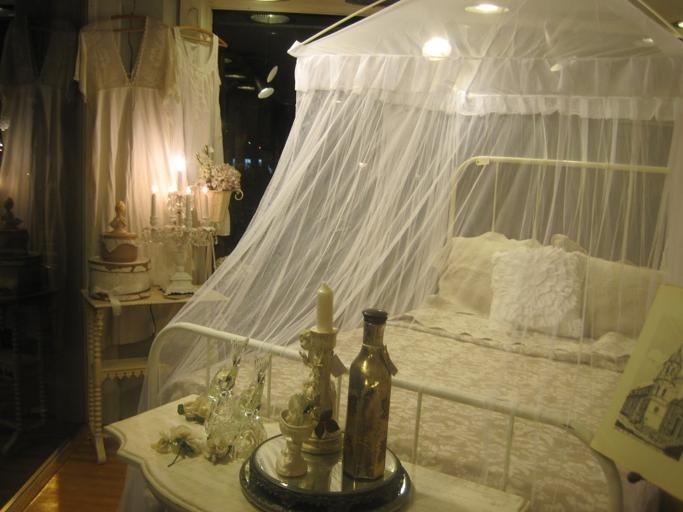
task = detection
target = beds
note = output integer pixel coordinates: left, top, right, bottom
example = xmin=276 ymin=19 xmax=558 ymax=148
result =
xmin=125 ymin=155 xmax=667 ymax=510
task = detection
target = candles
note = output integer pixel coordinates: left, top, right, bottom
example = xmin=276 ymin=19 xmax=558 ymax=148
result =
xmin=177 ymin=164 xmax=211 ymax=220
xmin=151 ymin=183 xmax=157 ymax=217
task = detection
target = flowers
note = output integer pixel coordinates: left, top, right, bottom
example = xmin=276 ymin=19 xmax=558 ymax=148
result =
xmin=146 ymin=422 xmax=205 ymax=467
xmin=195 ymin=144 xmax=242 ymax=192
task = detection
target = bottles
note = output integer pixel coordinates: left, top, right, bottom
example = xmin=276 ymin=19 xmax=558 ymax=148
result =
xmin=341 ymin=306 xmax=392 ymax=481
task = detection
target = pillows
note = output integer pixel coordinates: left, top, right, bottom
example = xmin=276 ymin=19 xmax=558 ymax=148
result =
xmin=440 ymin=230 xmax=664 ymax=346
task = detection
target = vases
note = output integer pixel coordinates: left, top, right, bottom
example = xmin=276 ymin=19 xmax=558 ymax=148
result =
xmin=209 ymin=188 xmax=231 ymax=223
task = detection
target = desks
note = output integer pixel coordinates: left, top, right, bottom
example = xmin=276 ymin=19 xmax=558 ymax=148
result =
xmin=101 ymin=393 xmax=531 ymax=512
xmin=78 ymin=282 xmax=229 ymax=466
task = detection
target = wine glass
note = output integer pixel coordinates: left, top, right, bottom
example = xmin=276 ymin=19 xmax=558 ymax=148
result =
xmin=274 ymin=407 xmax=321 ymax=478
